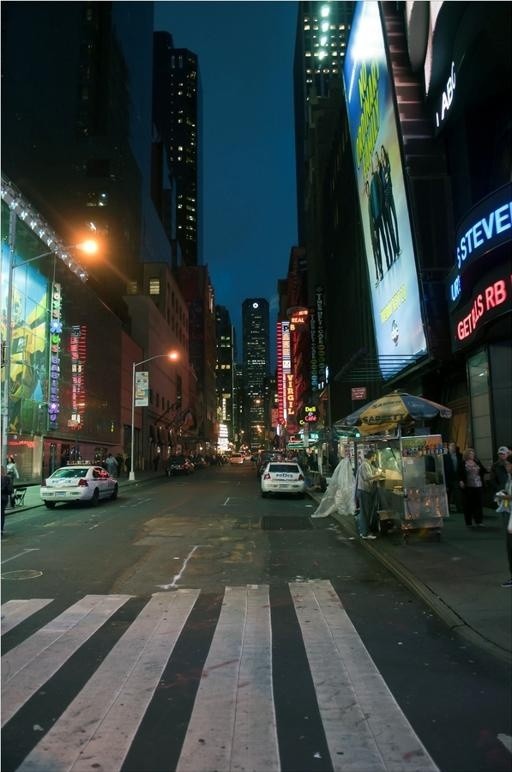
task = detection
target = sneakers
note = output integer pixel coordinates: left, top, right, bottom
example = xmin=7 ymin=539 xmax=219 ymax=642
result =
xmin=362 ymin=534 xmax=376 ymax=539
xmin=501 ymin=579 xmax=511 ymax=587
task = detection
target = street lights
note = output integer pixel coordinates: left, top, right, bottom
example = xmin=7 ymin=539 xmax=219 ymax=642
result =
xmin=2 ymin=207 xmax=100 ymax=469
xmin=127 ymin=350 xmax=179 ymax=483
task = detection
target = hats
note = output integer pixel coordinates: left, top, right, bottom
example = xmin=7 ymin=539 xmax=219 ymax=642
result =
xmin=497 ymin=447 xmax=509 ymax=455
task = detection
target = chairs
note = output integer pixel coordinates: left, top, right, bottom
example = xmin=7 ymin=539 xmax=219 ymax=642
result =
xmin=10 ymin=486 xmax=27 ymax=509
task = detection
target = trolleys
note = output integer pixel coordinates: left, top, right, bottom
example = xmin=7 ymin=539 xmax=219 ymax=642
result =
xmin=352 ymin=433 xmax=449 ymax=547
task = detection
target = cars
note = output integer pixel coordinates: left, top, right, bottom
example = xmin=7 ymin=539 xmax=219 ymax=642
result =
xmin=39 ymin=465 xmax=120 ymax=510
xmin=229 ymin=448 xmax=282 ymax=479
xmin=164 ymin=453 xmax=225 ymax=476
xmin=260 ymin=462 xmax=306 ymax=499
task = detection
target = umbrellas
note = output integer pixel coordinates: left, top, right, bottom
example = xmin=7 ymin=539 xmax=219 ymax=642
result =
xmin=334 ymin=390 xmax=453 ymax=437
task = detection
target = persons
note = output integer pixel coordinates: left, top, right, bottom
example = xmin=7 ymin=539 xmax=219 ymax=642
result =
xmin=360 ymin=146 xmax=402 ymax=285
xmin=445 ymin=442 xmax=511 ymax=589
xmin=355 ymin=451 xmax=382 ymax=540
xmin=1 ymin=465 xmax=12 ymax=533
xmin=7 ymin=457 xmax=19 ymax=493
xmin=287 ymin=449 xmax=317 ymax=471
xmin=102 ymin=453 xmax=131 ymax=477
